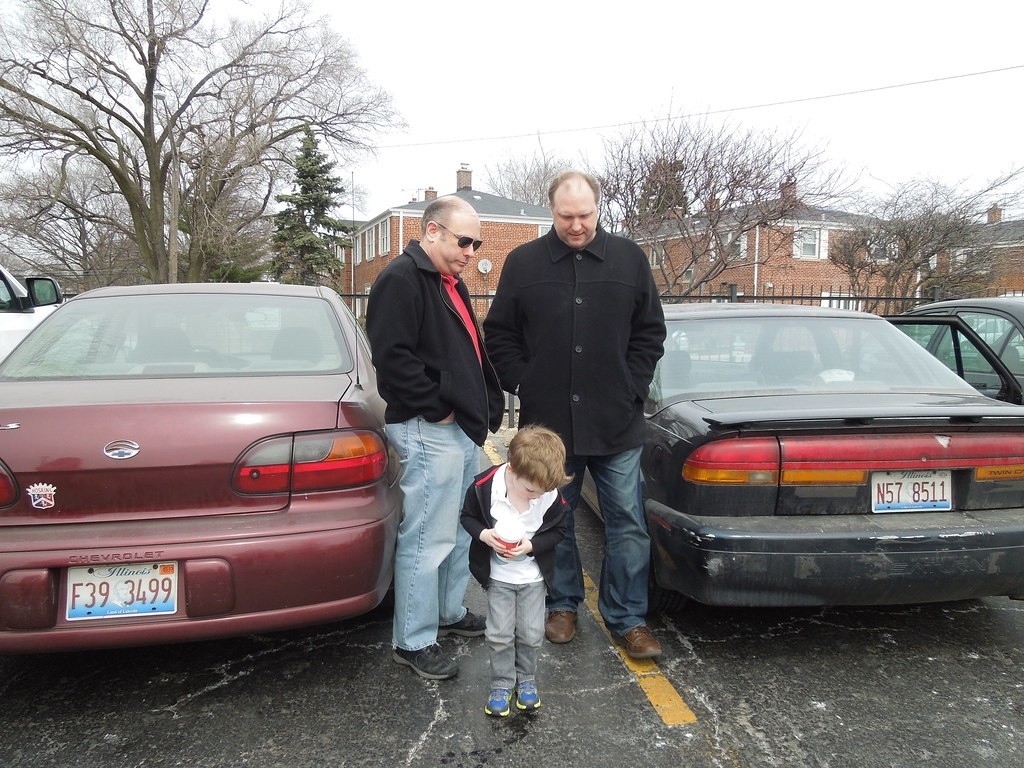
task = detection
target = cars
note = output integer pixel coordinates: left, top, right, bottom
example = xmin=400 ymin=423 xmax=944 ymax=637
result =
xmin=891 ymin=297 xmax=1024 ymax=405
xmin=921 ymin=311 xmax=1023 ymax=353
xmin=0 ymin=282 xmax=405 ymax=659
xmin=578 ymin=301 xmax=1024 ymax=622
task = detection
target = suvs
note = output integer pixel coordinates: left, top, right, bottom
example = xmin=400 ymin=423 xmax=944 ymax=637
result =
xmin=0 ymin=264 xmax=64 ymax=366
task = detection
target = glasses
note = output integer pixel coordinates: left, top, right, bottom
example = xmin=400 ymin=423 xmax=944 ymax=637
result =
xmin=436 ymin=221 xmax=483 ymax=252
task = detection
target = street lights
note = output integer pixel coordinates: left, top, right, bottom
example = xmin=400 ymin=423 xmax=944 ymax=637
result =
xmin=154 ymin=90 xmax=179 ymax=284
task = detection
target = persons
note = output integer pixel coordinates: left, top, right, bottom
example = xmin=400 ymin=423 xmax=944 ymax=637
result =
xmin=482 ymin=172 xmax=668 ymax=661
xmin=460 ymin=422 xmax=575 ymax=716
xmin=366 ymin=194 xmax=506 ymax=682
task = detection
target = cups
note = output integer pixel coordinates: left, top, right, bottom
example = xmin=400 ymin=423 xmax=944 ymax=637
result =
xmin=495 ymin=519 xmax=527 ymax=562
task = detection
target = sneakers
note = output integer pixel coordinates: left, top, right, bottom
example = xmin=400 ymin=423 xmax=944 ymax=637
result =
xmin=514 ymin=679 xmax=541 ymax=710
xmin=438 ymin=608 xmax=486 ymax=637
xmin=392 ymin=644 xmax=459 ymax=680
xmin=484 ymin=688 xmax=514 ymax=717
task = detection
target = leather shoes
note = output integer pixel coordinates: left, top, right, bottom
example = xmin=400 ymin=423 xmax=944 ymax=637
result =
xmin=545 ymin=611 xmax=578 ymax=643
xmin=611 ymin=626 xmax=662 ymax=658
